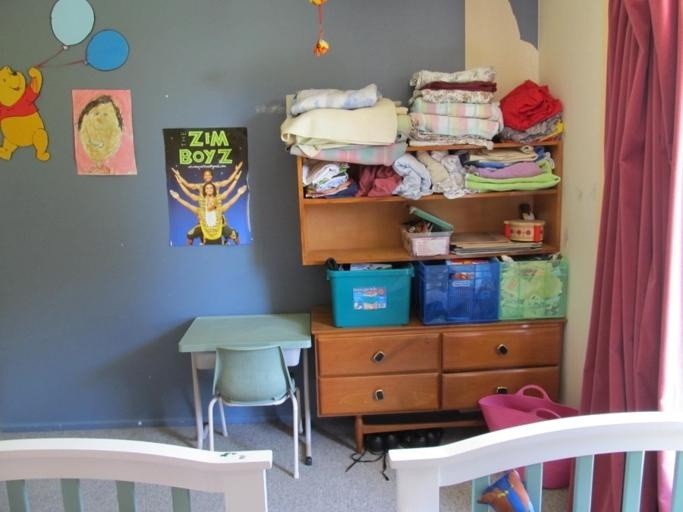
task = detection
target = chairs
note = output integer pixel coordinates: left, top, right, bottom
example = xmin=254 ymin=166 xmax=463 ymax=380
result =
xmin=207 ymin=347 xmax=301 ymax=478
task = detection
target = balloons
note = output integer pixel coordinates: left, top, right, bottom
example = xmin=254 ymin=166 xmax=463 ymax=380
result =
xmin=32 ymin=1 xmax=95 ymax=68
xmin=65 ymin=29 xmax=129 ymax=72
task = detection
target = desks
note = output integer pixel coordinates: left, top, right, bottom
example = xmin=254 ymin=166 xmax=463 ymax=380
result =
xmin=176 ymin=312 xmax=311 ymax=464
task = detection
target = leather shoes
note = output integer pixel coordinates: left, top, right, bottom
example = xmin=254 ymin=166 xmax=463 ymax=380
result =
xmin=370 ymin=429 xmax=444 ymax=456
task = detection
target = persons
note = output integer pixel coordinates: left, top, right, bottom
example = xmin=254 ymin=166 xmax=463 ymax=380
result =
xmin=168 ymin=184 xmax=247 ymax=245
xmin=77 ymin=92 xmax=124 ymax=175
xmin=172 ymin=170 xmax=240 ymax=245
xmin=170 ymin=160 xmax=243 ymax=197
